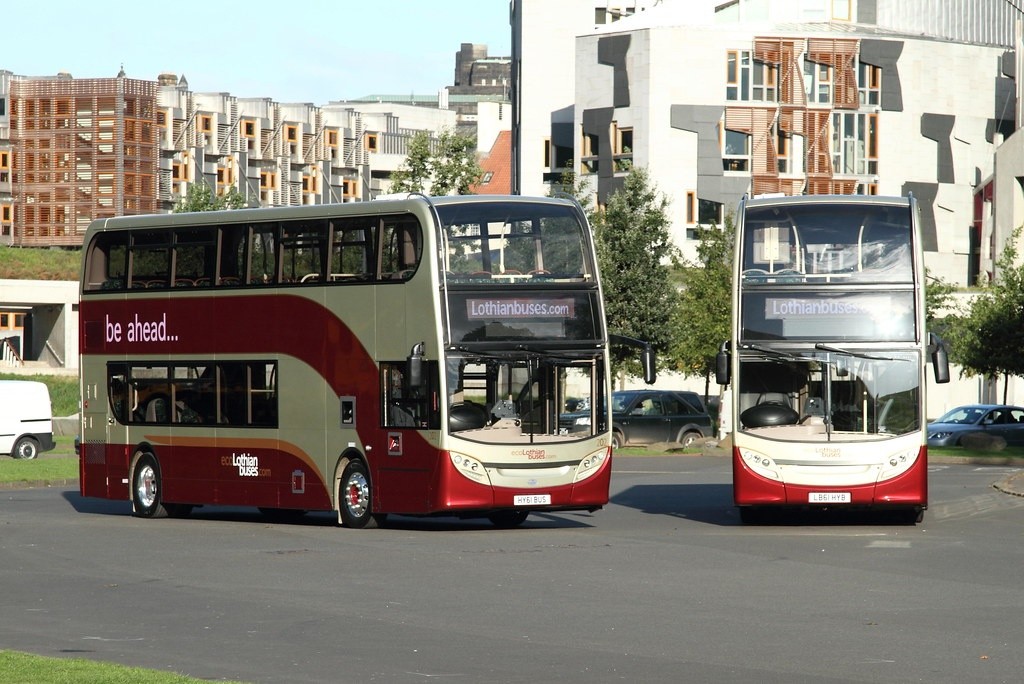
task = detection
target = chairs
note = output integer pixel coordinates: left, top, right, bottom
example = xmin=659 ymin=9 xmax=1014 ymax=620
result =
xmin=101 ymin=277 xmax=290 ymax=289
xmin=468 ymin=269 xmax=551 ymax=279
xmin=146 ymin=398 xmax=167 ymax=422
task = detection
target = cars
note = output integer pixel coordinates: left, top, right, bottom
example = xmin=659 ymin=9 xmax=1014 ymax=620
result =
xmin=927 ymin=404 xmax=1024 ymax=449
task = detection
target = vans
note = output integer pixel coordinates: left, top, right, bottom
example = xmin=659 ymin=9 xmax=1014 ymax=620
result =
xmin=0 ymin=379 xmax=53 ymax=460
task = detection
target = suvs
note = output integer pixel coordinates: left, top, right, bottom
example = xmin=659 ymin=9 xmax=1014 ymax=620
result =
xmin=557 ymin=390 xmax=713 ymax=451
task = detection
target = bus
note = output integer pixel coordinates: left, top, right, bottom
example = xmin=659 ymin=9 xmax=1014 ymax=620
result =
xmin=716 ymin=192 xmax=950 ymax=524
xmin=73 ymin=193 xmax=657 ymax=530
